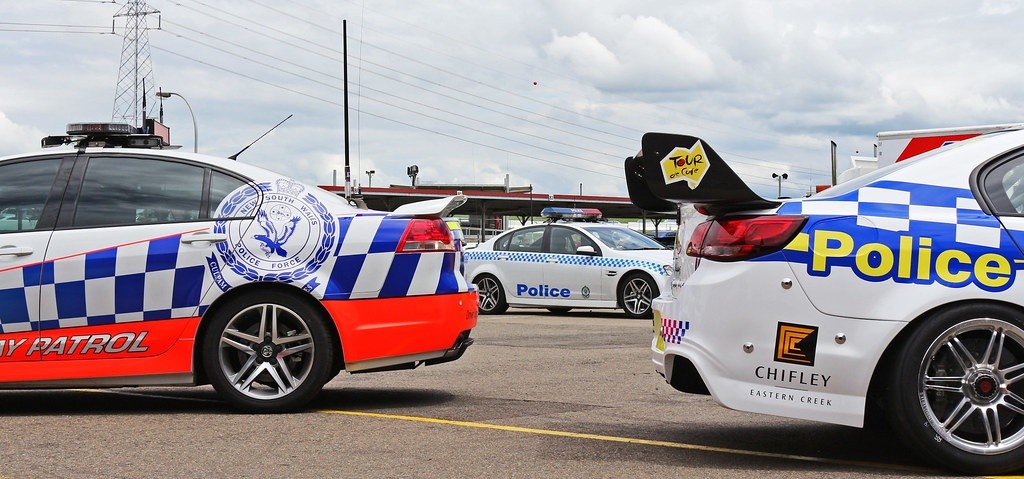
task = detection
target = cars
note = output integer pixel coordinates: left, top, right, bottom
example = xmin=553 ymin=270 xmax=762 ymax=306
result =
xmin=624 ymin=130 xmax=1024 ymax=479
xmin=0 ymin=116 xmax=480 ymax=414
xmin=463 ymin=207 xmax=674 ymax=319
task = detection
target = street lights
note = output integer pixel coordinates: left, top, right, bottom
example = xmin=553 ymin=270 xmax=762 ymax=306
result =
xmin=156 ymin=92 xmax=199 ymax=153
